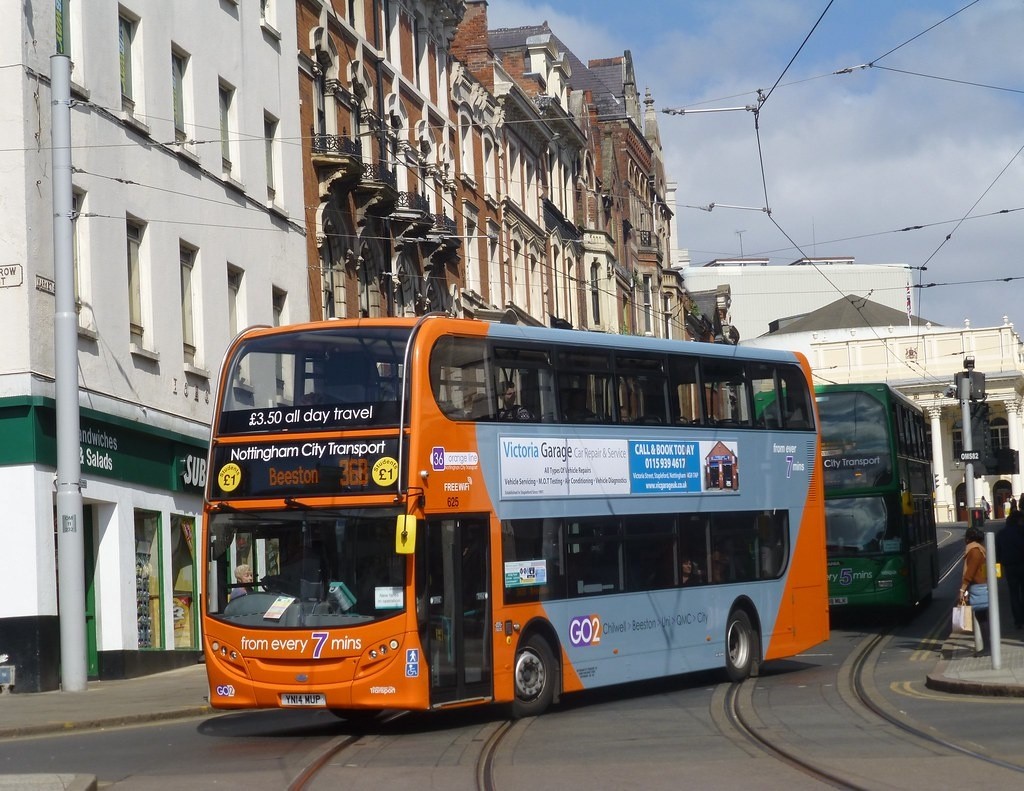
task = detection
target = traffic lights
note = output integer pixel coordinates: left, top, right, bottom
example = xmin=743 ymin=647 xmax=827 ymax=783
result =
xmin=970 ymin=506 xmax=985 ymax=528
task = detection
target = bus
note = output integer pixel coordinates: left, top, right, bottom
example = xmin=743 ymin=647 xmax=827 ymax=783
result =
xmin=201 ymin=310 xmax=831 ymax=726
xmin=752 ymin=382 xmax=940 ymax=618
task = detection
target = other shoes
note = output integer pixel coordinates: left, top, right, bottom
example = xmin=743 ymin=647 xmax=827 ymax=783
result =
xmin=973 ymin=650 xmax=990 ymax=657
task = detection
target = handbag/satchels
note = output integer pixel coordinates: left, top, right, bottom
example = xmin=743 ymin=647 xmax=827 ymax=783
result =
xmin=952 ymin=601 xmax=973 ymax=634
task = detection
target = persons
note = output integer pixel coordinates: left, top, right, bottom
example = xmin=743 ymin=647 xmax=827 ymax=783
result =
xmin=261 ymin=530 xmax=321 ymax=595
xmin=958 ymin=525 xmax=992 ymax=657
xmin=681 ymin=558 xmax=703 ymax=586
xmin=498 ymin=381 xmax=530 ymax=421
xmin=995 ymin=493 xmax=1024 ymax=628
xmin=981 ymin=496 xmax=990 ymax=520
xmin=230 ymin=565 xmax=253 ymax=602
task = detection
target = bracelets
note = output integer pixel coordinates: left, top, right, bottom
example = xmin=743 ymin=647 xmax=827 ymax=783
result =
xmin=960 ymin=589 xmax=965 ymax=592
